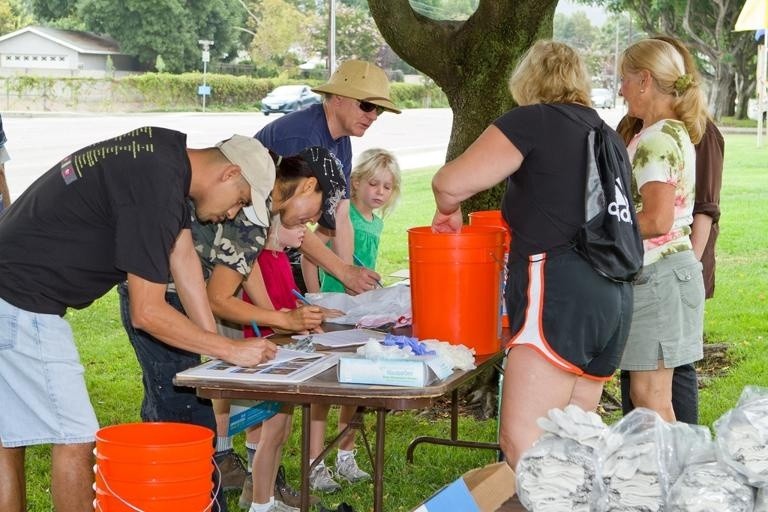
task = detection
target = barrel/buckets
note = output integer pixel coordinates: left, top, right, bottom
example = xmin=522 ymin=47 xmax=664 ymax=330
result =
xmin=92 ymin=422 xmax=222 ymax=512
xmin=407 ymin=224 xmax=507 ymax=356
xmin=467 ymin=207 xmax=512 ymax=331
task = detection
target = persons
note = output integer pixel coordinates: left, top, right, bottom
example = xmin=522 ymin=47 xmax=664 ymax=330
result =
xmin=429 ymin=38 xmax=643 ymax=474
xmin=613 ymin=36 xmax=726 ymax=425
xmin=194 ymin=147 xmax=402 ymax=512
xmin=609 ymin=37 xmax=709 ymax=431
xmin=110 ymin=146 xmax=340 ymax=511
xmin=0 ymin=124 xmax=275 ymax=511
xmin=252 ymin=57 xmax=422 ymax=301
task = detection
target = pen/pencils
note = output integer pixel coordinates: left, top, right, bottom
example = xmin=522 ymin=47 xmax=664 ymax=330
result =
xmin=292 ymin=288 xmax=311 ymax=305
xmin=352 ymin=255 xmax=384 ymax=289
xmin=250 ymin=319 xmax=262 ymax=336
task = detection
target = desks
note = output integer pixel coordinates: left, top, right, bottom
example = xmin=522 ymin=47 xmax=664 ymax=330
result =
xmin=172 ymin=322 xmax=504 ymax=512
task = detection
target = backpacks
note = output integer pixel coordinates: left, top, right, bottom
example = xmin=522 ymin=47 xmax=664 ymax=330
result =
xmin=531 ymin=102 xmax=650 ymax=285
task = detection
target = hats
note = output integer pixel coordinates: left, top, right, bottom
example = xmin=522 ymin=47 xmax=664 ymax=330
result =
xmin=310 ymin=56 xmax=403 ymax=114
xmin=296 ymin=145 xmax=348 ymax=231
xmin=215 ymin=133 xmax=276 ymax=227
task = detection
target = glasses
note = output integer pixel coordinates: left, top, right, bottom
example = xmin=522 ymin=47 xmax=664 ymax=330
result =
xmin=356 ymin=100 xmax=385 ymax=116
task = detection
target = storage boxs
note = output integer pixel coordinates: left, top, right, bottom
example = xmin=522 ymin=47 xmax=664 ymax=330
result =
xmin=409 ymin=476 xmax=482 ymax=512
xmin=459 ymin=459 xmax=526 ymax=511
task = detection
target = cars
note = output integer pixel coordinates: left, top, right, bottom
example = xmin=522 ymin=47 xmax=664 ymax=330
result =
xmin=590 ymin=88 xmax=614 ymax=110
xmin=259 ymin=84 xmax=320 ymax=116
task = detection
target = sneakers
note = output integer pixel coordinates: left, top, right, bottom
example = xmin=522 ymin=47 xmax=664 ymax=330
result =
xmin=334 ymin=450 xmax=370 ymax=484
xmin=236 ymin=468 xmax=321 ymax=509
xmin=210 ymin=447 xmax=248 ymax=491
xmin=302 ymin=456 xmax=341 ymax=494
xmin=247 ymin=496 xmax=300 ymax=511
